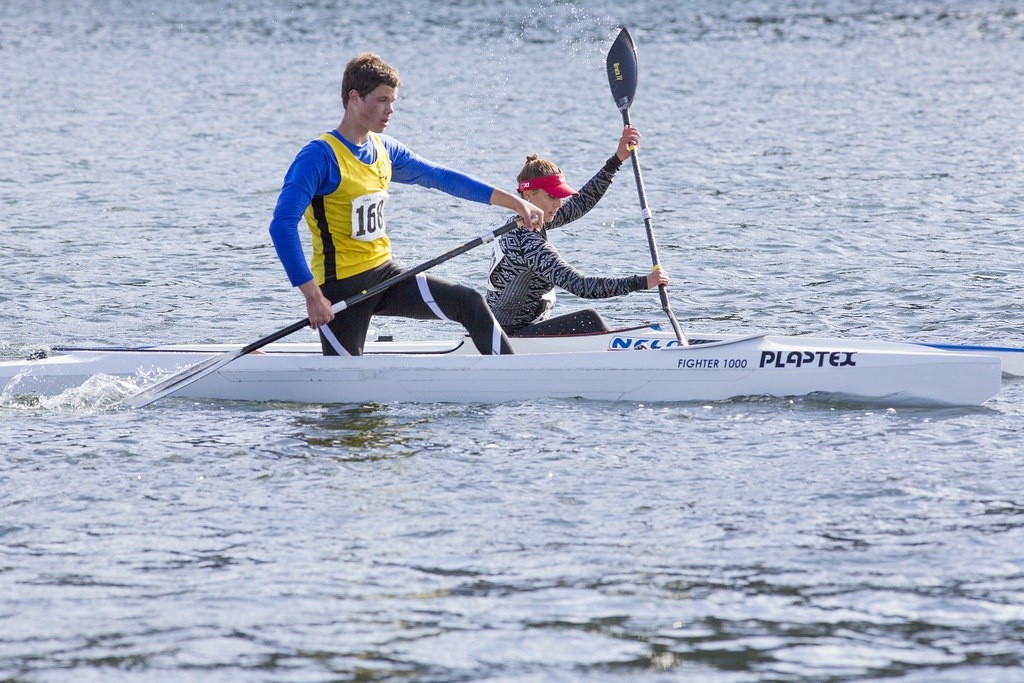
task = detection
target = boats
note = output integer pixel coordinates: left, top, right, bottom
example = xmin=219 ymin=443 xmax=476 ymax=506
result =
xmin=0 ymin=330 xmax=1002 ymax=406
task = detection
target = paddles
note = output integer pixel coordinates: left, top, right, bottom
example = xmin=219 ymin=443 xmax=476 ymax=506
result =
xmin=123 ymin=215 xmax=541 ymax=409
xmin=607 ymin=26 xmax=691 ymax=349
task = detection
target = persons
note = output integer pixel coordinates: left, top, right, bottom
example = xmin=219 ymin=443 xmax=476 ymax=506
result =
xmin=487 ymin=123 xmax=669 ymax=338
xmin=269 ymin=52 xmax=544 ymax=355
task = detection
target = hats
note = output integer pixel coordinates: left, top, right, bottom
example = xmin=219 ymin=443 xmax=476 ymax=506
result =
xmin=517 ymin=173 xmax=580 ymax=198
